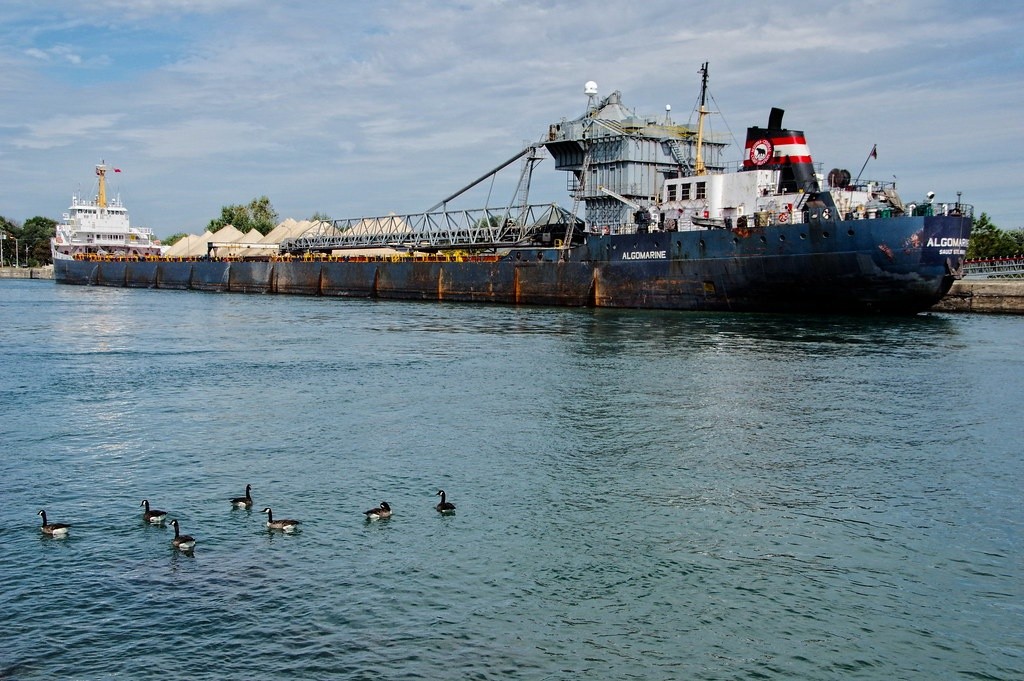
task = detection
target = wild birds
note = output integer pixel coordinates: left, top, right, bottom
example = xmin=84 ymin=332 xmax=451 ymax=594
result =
xmin=436 ymin=490 xmax=456 ymax=513
xmin=229 ymin=484 xmax=253 ymax=507
xmin=37 ymin=510 xmax=73 ymax=534
xmin=169 ymin=520 xmax=195 ymax=548
xmin=140 ymin=500 xmax=168 ymax=523
xmin=259 ymin=508 xmax=302 ymax=530
xmin=363 ymin=502 xmax=393 ymax=519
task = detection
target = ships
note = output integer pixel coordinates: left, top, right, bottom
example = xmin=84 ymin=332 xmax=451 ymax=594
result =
xmin=49 ymin=61 xmax=977 ymax=319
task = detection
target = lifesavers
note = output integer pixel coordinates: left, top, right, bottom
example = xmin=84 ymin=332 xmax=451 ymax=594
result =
xmin=602 ymin=225 xmax=610 ymax=234
xmin=779 ymin=212 xmax=788 ymax=222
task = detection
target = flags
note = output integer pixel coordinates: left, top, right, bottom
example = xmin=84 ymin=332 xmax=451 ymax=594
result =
xmin=872 ymin=147 xmax=878 ymax=159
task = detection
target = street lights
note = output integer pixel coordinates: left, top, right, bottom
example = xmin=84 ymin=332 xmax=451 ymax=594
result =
xmin=26 ymin=243 xmax=32 ymax=265
xmin=10 ymin=237 xmax=18 ymax=266
xmin=1 ymin=231 xmax=7 ymax=267
